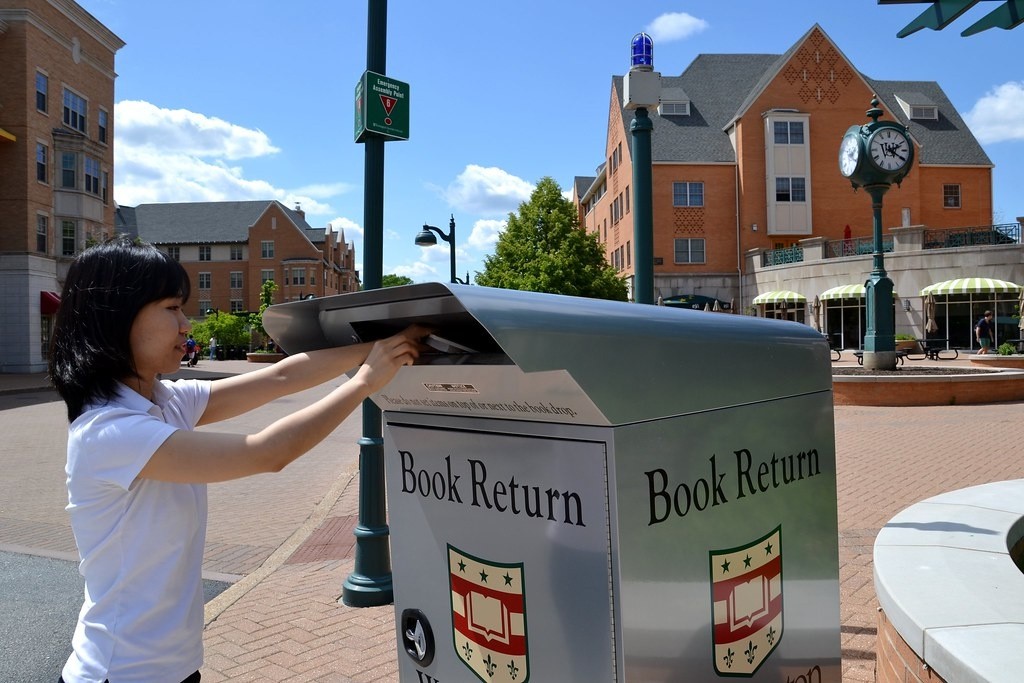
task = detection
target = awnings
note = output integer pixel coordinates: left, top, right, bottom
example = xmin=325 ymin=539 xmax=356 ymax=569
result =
xmin=752 ymin=290 xmax=805 ymax=304
xmin=919 ymin=277 xmax=1024 ymax=297
xmin=819 ymin=283 xmax=897 ymax=300
xmin=40 ymin=290 xmax=65 ymax=314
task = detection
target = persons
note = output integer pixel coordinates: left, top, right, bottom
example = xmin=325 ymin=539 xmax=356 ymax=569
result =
xmin=185 ymin=334 xmax=198 ymax=368
xmin=209 ymin=333 xmax=218 ymax=361
xmin=46 ymin=234 xmax=440 ymax=683
xmin=976 ymin=309 xmax=994 ymax=355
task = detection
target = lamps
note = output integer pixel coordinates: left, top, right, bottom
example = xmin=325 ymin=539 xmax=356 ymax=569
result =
xmin=809 ymin=304 xmax=813 ymax=313
xmin=903 ymin=299 xmax=910 ymax=311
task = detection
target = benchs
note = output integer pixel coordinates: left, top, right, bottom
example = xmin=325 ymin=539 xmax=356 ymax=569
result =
xmin=896 ymin=347 xmax=926 ymax=366
xmin=831 ymin=348 xmax=844 ymax=362
xmin=939 ymin=346 xmax=963 ymax=359
xmin=854 ymin=351 xmax=863 ymax=365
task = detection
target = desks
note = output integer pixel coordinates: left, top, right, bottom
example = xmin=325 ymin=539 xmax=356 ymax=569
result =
xmin=1007 ymin=339 xmax=1024 ymax=354
xmin=915 ymin=339 xmax=950 ymax=361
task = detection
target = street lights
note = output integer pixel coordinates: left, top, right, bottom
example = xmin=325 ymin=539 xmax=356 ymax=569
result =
xmin=299 ymin=290 xmax=314 ymax=301
xmin=414 ymin=213 xmax=455 ymax=284
xmin=206 ymin=306 xmax=220 ymax=345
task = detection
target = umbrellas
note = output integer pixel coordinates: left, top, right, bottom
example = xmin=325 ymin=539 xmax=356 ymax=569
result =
xmin=1017 ymin=284 xmax=1024 ymax=332
xmin=812 ymin=294 xmax=822 ymax=334
xmin=703 ymin=302 xmax=712 ymax=312
xmin=658 ymin=294 xmax=665 ymax=306
xmin=923 ymin=292 xmax=937 ymax=359
xmin=779 ymin=299 xmax=789 ymax=320
xmin=712 ymin=299 xmax=724 ymax=313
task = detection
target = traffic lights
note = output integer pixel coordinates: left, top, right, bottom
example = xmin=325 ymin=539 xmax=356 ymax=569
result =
xmin=630 ymin=32 xmax=654 ymax=71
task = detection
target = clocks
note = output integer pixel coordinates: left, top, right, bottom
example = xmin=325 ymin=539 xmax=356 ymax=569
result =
xmin=838 ymin=135 xmax=859 ymax=176
xmin=870 ymin=128 xmax=910 ymax=171
